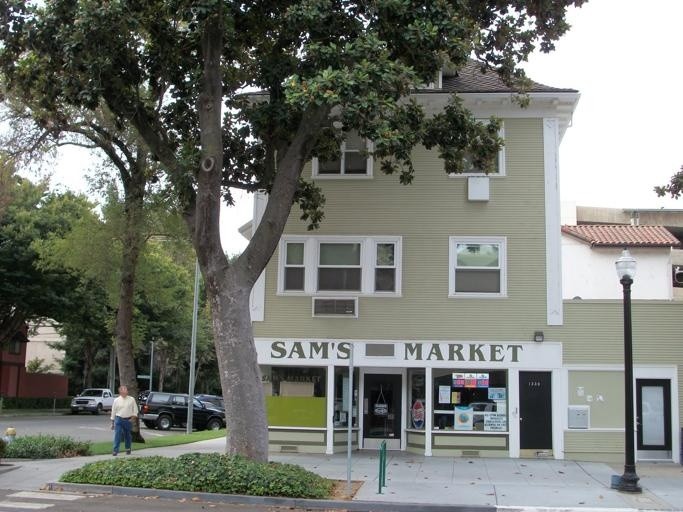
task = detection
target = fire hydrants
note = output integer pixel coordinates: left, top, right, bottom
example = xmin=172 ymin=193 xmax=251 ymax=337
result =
xmin=5 ymin=426 xmax=16 ymax=444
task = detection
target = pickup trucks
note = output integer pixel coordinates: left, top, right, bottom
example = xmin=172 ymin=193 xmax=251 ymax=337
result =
xmin=70 ymin=388 xmax=120 ymax=415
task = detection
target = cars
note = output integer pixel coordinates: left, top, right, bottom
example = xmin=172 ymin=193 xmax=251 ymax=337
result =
xmin=136 ymin=390 xmax=226 ymax=431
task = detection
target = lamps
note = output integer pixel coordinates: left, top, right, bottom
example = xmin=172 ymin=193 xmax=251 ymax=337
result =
xmin=533 ymin=331 xmax=544 ymax=342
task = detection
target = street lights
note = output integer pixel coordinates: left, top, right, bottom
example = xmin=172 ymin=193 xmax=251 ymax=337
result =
xmin=149 ymin=336 xmax=158 ymax=391
xmin=614 ymin=249 xmax=642 ymax=493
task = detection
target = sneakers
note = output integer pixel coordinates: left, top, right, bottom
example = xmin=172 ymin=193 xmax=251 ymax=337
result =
xmin=112 ymin=449 xmax=131 ymax=456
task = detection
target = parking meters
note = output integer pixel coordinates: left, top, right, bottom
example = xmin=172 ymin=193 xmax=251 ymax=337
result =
xmin=53 ymin=392 xmax=57 ymax=415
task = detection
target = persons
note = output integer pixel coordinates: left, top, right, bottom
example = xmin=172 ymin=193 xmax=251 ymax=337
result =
xmin=109 ymin=384 xmax=138 ymax=456
